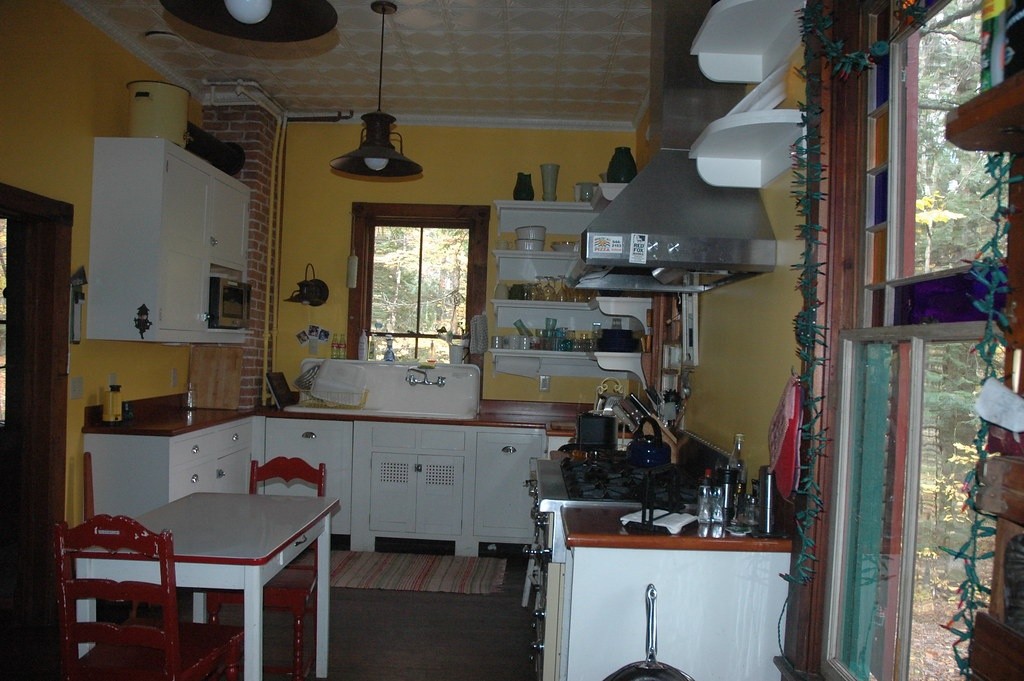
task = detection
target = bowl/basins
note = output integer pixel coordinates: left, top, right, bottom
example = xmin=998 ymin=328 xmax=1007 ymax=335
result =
xmin=599 ymin=172 xmax=608 ymax=183
xmin=515 ymin=226 xmax=546 ymax=251
xmin=551 ymin=241 xmax=575 ymax=252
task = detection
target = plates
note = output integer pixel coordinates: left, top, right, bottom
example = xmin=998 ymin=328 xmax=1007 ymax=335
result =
xmin=597 ymin=329 xmax=638 ymax=352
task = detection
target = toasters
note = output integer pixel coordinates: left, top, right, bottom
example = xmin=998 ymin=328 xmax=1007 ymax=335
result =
xmin=576 ymin=410 xmax=618 ymax=451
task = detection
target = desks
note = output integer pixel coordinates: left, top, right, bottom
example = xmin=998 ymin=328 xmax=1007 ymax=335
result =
xmin=67 ymin=492 xmax=340 ymax=681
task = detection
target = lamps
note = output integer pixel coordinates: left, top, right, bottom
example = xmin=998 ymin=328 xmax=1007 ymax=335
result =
xmin=330 ymin=1 xmax=422 ymax=178
xmin=161 ymin=0 xmax=338 ymax=42
xmin=283 ymin=263 xmax=329 ymax=308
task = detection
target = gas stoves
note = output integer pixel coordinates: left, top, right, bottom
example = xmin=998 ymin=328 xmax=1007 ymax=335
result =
xmin=535 ymin=462 xmax=721 ymax=512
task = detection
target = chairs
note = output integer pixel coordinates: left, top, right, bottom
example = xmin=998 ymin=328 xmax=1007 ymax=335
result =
xmin=205 ymin=456 xmax=326 ymax=681
xmin=50 ymin=512 xmax=243 ymax=681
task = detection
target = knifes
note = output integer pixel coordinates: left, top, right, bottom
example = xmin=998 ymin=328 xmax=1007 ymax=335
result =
xmin=612 ymin=392 xmax=651 ymax=432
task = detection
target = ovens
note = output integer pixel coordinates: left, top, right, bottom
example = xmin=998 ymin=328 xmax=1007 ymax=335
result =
xmin=525 ymin=474 xmax=561 ymax=681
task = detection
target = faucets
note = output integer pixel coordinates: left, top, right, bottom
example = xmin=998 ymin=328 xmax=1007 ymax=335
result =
xmin=405 ymin=367 xmax=446 ymax=388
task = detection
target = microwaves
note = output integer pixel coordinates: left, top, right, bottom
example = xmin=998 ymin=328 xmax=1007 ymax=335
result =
xmin=209 ymin=277 xmax=251 ymax=330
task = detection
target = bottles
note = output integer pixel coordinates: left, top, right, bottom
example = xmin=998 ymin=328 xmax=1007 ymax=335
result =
xmin=183 ymin=383 xmax=196 ymax=409
xmin=699 ymin=434 xmax=784 ymax=538
xmin=575 ymin=322 xmax=602 ymax=351
xmin=513 ymin=173 xmax=534 ymax=200
xmin=331 ymin=333 xmax=338 ymax=359
xmin=104 ymin=385 xmax=122 ymax=421
xmin=606 ymin=147 xmax=638 ymax=183
xmin=338 ymin=334 xmax=346 ymax=358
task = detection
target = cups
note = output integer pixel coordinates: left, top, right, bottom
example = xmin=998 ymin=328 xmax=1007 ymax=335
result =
xmin=491 ymin=318 xmax=575 ymax=351
xmin=494 ymin=284 xmax=537 ymax=300
xmin=450 ymin=345 xmax=470 ymax=364
xmin=540 ymin=164 xmax=560 ymax=202
xmin=495 ymin=240 xmax=513 ymax=250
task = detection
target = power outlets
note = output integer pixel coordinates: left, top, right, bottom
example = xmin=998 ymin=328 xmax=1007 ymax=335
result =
xmin=540 ymin=376 xmax=551 ymax=392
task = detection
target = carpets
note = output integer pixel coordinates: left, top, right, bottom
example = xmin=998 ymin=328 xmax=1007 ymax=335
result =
xmin=300 ymin=550 xmax=507 ymax=594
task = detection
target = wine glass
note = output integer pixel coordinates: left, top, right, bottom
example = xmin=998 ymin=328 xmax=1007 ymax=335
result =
xmin=532 ymin=275 xmax=585 ymax=302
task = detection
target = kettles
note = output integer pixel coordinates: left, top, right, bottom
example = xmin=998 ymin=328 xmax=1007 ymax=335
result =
xmin=626 ymin=417 xmax=672 ymax=468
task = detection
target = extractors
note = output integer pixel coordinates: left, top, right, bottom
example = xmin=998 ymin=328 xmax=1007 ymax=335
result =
xmin=565 ymin=0 xmax=777 ymax=293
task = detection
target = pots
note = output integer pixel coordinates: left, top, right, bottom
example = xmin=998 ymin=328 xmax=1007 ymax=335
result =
xmin=604 ymin=584 xmax=694 ymax=681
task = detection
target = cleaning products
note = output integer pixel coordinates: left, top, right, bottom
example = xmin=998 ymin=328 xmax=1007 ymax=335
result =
xmin=367 ymin=334 xmax=377 ymax=361
xmin=426 ymin=340 xmax=437 ymax=363
xmin=384 ymin=335 xmax=394 ymax=362
xmin=358 ymin=329 xmax=367 ymax=360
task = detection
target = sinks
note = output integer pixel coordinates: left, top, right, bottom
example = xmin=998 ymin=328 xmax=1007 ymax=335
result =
xmin=376 ymin=411 xmax=457 ymax=415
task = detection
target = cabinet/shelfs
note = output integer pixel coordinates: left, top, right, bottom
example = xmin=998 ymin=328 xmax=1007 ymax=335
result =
xmin=491 ymin=199 xmax=652 ymax=389
xmin=688 ymin=1 xmax=809 ymax=190
xmin=82 ymin=416 xmax=791 ymax=681
xmin=84 ymin=136 xmax=250 ymax=341
